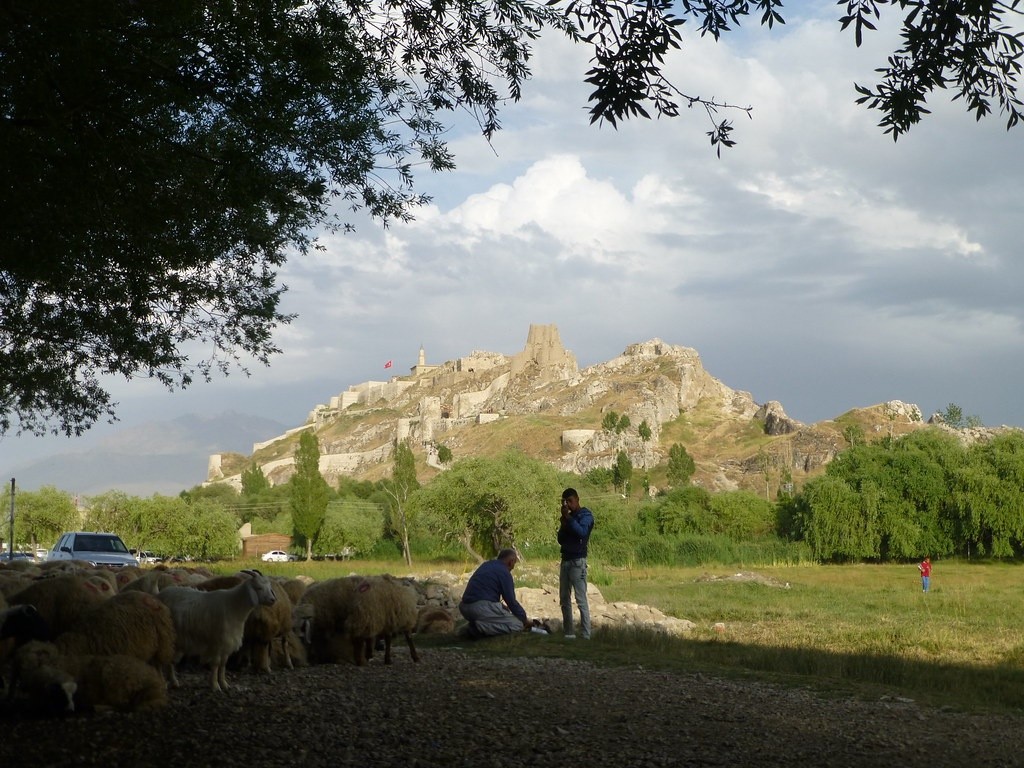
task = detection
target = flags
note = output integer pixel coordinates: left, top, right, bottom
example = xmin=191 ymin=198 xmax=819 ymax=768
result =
xmin=384 ymin=360 xmax=391 ymax=369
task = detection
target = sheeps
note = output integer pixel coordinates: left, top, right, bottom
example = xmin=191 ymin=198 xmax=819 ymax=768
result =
xmin=0 ymin=557 xmax=420 ymax=714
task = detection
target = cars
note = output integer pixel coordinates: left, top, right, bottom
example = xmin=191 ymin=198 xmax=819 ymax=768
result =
xmin=0 ymin=548 xmax=49 ymax=562
xmin=136 ymin=550 xmax=162 ymax=564
xmin=47 ymin=533 xmax=139 ymax=568
xmin=262 ymin=550 xmax=298 ymax=562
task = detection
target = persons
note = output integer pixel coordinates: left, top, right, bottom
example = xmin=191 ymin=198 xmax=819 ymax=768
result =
xmin=556 ymin=488 xmax=594 ymax=639
xmin=459 ymin=548 xmax=536 ymax=640
xmin=918 ymin=557 xmax=933 ymax=590
